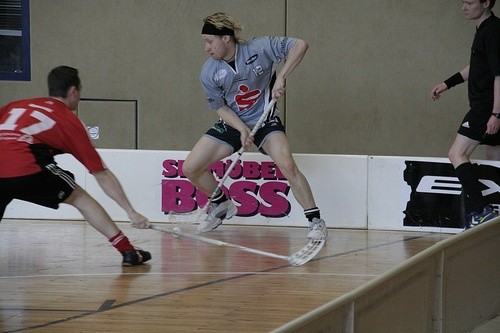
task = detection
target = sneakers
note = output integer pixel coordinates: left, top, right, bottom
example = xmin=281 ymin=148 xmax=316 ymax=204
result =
xmin=461 ymin=205 xmax=498 ymax=233
xmin=122 ymin=250 xmax=152 ymax=267
xmin=307 ymin=217 xmax=328 ymax=240
xmin=196 ymin=197 xmax=238 ymax=232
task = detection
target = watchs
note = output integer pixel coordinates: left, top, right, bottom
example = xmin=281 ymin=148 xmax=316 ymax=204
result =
xmin=491 ymin=113 xmax=500 ymax=120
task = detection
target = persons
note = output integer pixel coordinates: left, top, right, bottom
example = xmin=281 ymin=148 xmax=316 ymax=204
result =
xmin=0 ymin=65 xmax=152 ymax=267
xmin=432 ymin=0 xmax=500 ymax=233
xmin=183 ymin=12 xmax=327 ymax=240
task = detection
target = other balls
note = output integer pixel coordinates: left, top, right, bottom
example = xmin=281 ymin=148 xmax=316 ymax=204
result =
xmin=172 ymin=227 xmax=181 ymax=237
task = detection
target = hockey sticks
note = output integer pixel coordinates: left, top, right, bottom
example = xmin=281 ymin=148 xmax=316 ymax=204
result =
xmin=168 ymin=99 xmax=275 ymax=224
xmin=150 ymin=225 xmax=326 ymax=267
